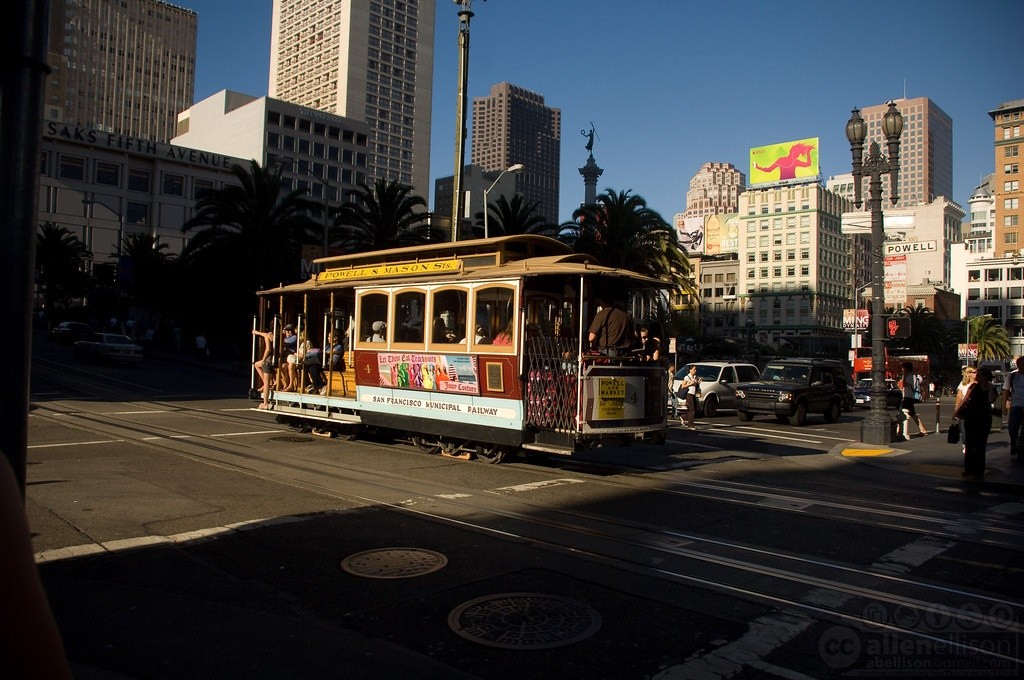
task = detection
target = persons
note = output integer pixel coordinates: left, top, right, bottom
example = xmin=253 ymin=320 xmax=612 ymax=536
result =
xmin=37 ymin=308 xmax=43 ymax=320
xmin=252 ymin=291 xmax=660 ymax=408
xmin=894 ymin=378 xmax=953 ymax=401
xmin=898 ymin=362 xmax=929 ymax=440
xmin=680 ymin=365 xmax=702 ymax=431
xmin=581 ymin=122 xmax=595 ymax=154
xmin=1001 ymin=356 xmax=1024 ymax=463
xmin=665 ymin=363 xmax=678 ymax=420
xmin=87 ymin=312 xmax=207 ymax=362
xmin=0 ymin=451 xmax=72 ymax=680
xmin=953 ymin=365 xmax=998 ymax=493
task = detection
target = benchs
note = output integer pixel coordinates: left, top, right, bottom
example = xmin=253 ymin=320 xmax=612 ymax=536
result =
xmin=270 ymin=348 xmax=358 ymax=400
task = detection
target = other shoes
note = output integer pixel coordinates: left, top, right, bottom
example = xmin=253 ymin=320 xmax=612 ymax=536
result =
xmin=961 ymin=471 xmax=975 ymax=477
xmin=307 ymin=384 xmax=318 ymax=394
xmin=688 ymin=427 xmax=696 ymax=430
xmin=680 ymin=416 xmax=685 ymax=424
xmin=1010 ymin=447 xmax=1015 ymax=456
xmin=319 ymin=385 xmax=326 ymax=395
xmin=258 ymin=385 xmax=264 ymax=391
xmin=283 ymin=386 xmax=294 ymax=392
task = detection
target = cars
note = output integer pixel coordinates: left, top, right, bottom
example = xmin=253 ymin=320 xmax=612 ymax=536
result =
xmin=854 ymin=377 xmax=904 ymax=410
xmin=71 ymin=331 xmax=145 ymax=365
xmin=47 ymin=321 xmax=94 ymax=345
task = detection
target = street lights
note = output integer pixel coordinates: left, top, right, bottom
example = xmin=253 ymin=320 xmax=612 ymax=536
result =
xmin=80 ymin=198 xmax=124 ymax=260
xmin=451 ymin=0 xmax=478 ymax=241
xmin=966 ymin=311 xmax=992 ymax=366
xmin=483 ymin=163 xmax=526 ymax=239
xmin=844 ymin=100 xmax=904 ymax=445
xmin=273 ymin=155 xmax=330 ymax=258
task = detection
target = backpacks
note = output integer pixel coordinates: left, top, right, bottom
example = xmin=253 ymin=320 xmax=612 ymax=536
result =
xmin=908 ymin=372 xmax=923 ymax=400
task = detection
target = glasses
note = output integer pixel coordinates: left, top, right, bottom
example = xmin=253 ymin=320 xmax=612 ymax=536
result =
xmin=642 ymin=336 xmax=647 ymax=340
xmin=970 ymin=372 xmax=976 ymax=374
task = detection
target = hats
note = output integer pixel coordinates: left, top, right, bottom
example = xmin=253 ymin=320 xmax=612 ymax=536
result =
xmin=283 ymin=324 xmax=294 ymax=330
xmin=372 ymin=320 xmax=387 ymax=331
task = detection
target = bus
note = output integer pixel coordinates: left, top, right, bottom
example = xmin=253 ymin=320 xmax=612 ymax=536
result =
xmin=247 ymin=231 xmax=675 ymax=464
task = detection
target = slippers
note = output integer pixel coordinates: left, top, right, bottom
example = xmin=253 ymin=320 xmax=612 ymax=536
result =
xmin=917 ymin=432 xmax=929 ymax=437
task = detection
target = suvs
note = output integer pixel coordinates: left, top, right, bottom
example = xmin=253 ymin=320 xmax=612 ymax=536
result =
xmin=734 ymin=357 xmax=849 ymax=427
xmin=667 ymin=361 xmax=761 ymax=418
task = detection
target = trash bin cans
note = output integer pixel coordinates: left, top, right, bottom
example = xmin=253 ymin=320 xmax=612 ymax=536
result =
xmin=990 ymin=382 xmax=1004 ymax=431
xmin=945 ymin=387 xmax=954 ymax=397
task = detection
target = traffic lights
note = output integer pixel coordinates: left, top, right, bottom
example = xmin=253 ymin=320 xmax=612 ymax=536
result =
xmin=886 ymin=316 xmax=912 ymax=340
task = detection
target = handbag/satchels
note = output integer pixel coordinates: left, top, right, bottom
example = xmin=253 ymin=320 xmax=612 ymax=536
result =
xmin=333 ymin=358 xmax=346 ymax=372
xmin=947 ymin=423 xmax=961 ymax=445
xmin=676 ymin=384 xmax=688 ymax=400
xmin=695 ymin=379 xmax=701 ymax=398
xmin=304 ymin=349 xmax=322 ymax=365
xmin=958 ymin=405 xmax=968 ymax=420
